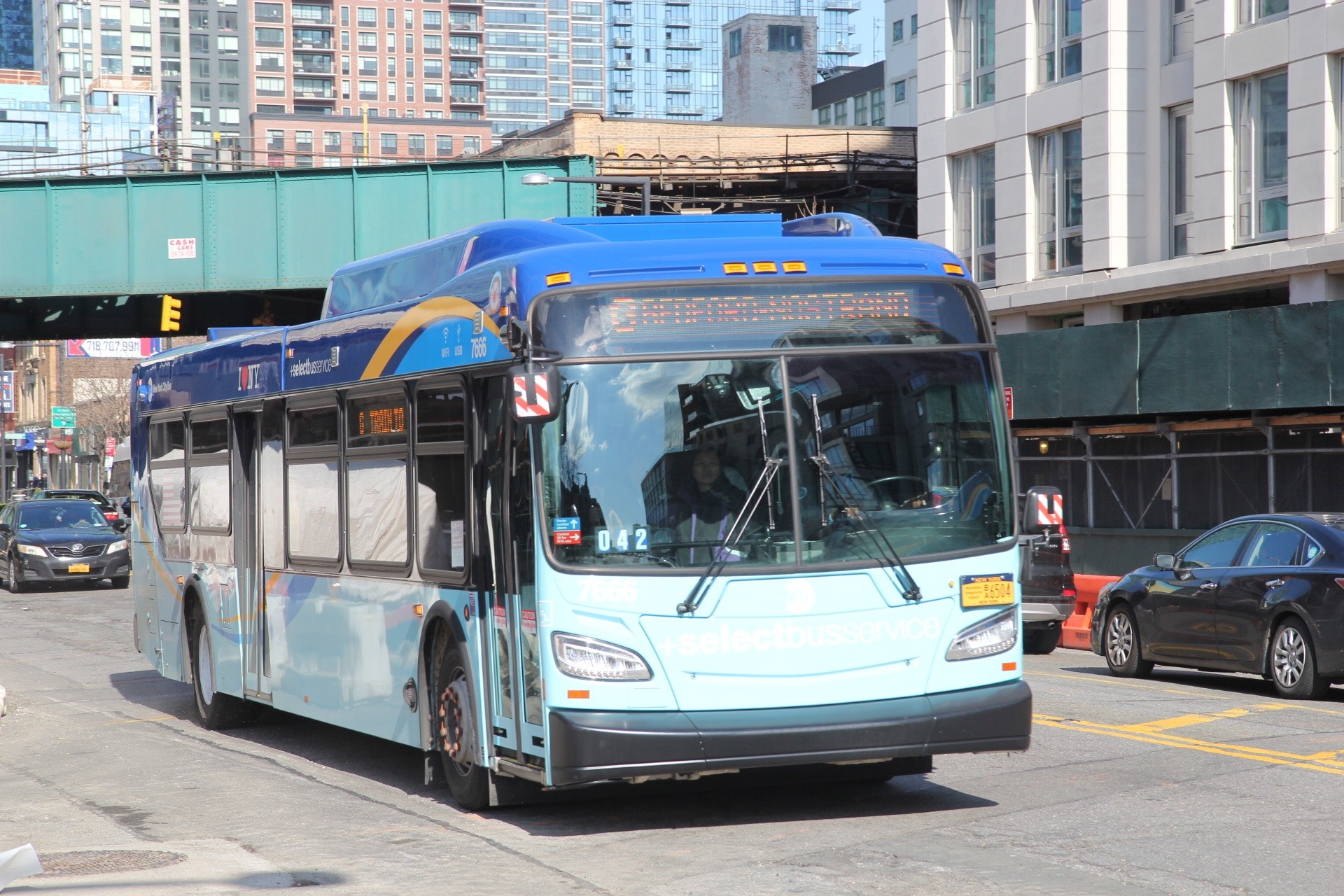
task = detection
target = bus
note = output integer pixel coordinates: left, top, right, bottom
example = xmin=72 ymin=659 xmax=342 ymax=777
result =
xmin=125 ymin=208 xmax=1035 ymax=812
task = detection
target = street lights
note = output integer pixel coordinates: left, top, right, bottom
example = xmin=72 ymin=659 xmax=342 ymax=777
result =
xmin=0 ymin=342 xmax=65 ymax=498
xmin=520 ymin=172 xmax=651 ymax=216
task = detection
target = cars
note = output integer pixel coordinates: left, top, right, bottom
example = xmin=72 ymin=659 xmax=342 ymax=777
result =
xmin=0 ymin=487 xmax=133 ymax=594
xmin=1087 ymin=509 xmax=1344 ymax=702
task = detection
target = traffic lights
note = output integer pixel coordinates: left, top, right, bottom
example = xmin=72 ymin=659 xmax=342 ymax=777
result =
xmin=160 ymin=295 xmax=182 ymax=332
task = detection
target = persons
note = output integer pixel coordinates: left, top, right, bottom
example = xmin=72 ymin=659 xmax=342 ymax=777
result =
xmin=665 ymin=447 xmax=754 ymax=565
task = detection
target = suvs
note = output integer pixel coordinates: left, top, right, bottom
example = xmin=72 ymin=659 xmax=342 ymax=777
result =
xmin=929 ymin=482 xmax=1078 ymax=655
